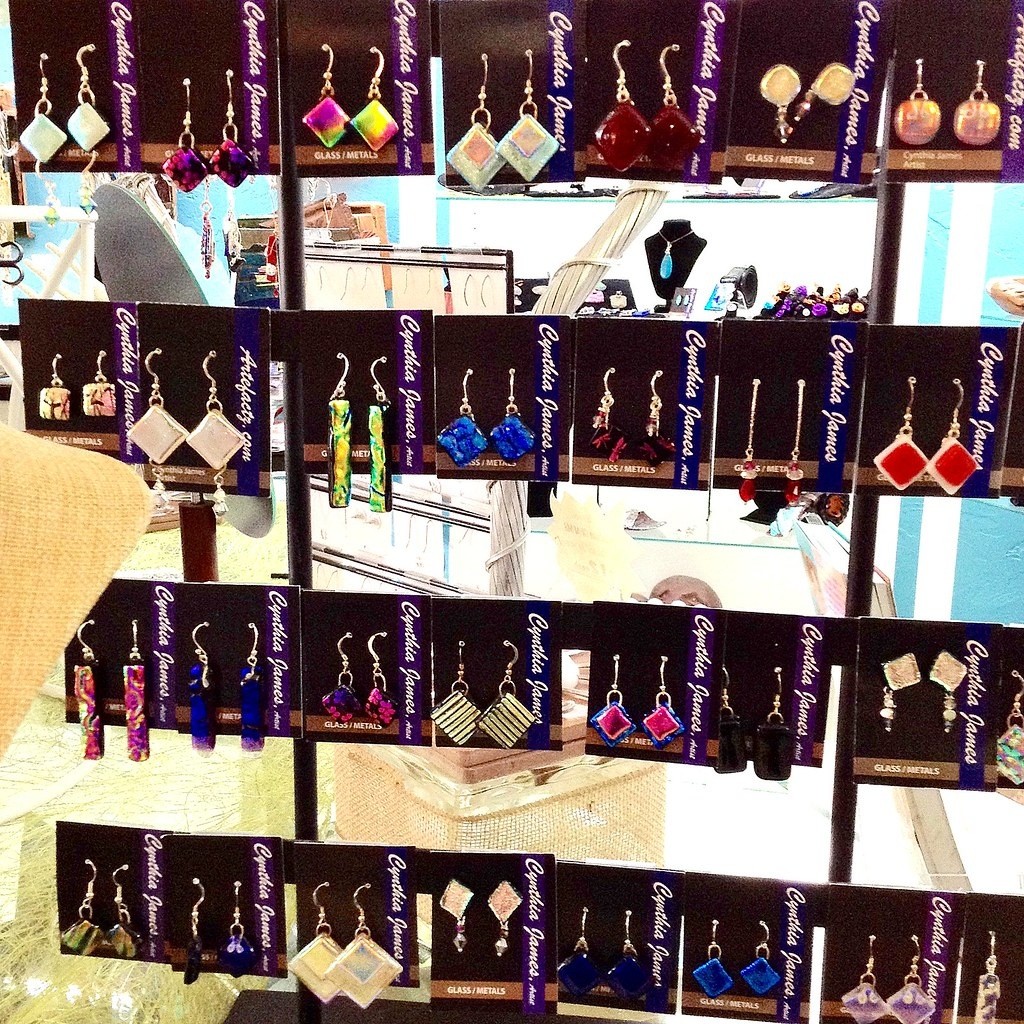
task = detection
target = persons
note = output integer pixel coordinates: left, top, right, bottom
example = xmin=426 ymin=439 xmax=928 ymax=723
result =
xmin=644 ymin=219 xmax=707 ymax=298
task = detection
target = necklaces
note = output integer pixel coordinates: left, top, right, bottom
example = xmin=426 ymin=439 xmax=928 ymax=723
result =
xmin=659 ymin=227 xmax=694 ymax=278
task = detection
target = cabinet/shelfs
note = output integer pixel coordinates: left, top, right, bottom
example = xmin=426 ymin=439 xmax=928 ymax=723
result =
xmin=16 ymin=135 xmax=1024 ymax=1024
